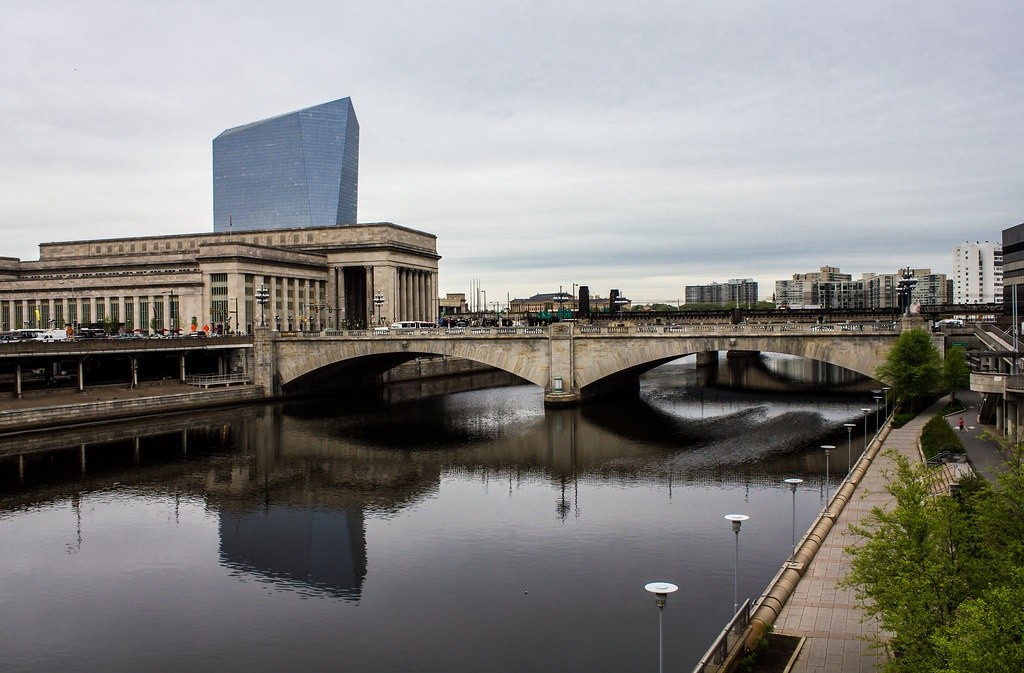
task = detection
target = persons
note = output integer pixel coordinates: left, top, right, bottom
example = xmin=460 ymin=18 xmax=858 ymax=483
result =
xmin=67 ymin=324 xmax=73 ymax=338
xmin=959 ymin=417 xmax=964 ymax=432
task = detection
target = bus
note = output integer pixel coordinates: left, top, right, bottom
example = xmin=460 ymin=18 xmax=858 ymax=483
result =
xmin=397 ymin=321 xmax=440 ymax=330
xmin=953 ymin=315 xmax=997 ymax=323
xmin=663 ymin=326 xmax=686 ymax=333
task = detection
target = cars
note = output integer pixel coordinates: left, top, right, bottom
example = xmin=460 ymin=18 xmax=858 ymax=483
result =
xmin=812 ymin=326 xmax=834 ymax=332
xmin=190 ymin=331 xmax=207 ymax=338
xmin=37 ymin=334 xmax=53 ymax=338
xmin=526 ymin=328 xmax=543 ymax=334
xmin=446 ymin=327 xmax=465 ymax=334
xmin=739 ymin=322 xmax=746 ymax=325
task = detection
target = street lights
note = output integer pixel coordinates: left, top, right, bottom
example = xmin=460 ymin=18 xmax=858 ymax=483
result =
xmin=820 ymin=304 xmax=832 ymax=324
xmin=490 ymin=302 xmax=499 ymax=321
xmin=965 ymin=303 xmax=967 ymax=314
xmin=482 ymin=291 xmax=486 ymax=310
xmin=894 ymin=267 xmax=917 ymax=316
xmin=162 ymin=290 xmax=174 ymax=339
xmin=573 ymin=283 xmax=579 ymax=327
xmin=785 ymin=388 xmax=891 ymax=564
xmin=254 ymin=283 xmax=270 ymax=327
xmin=644 ymin=582 xmax=678 ymax=673
xmin=59 ymin=281 xmax=75 ymax=338
xmin=615 ymin=292 xmax=629 ymax=323
xmin=373 ymin=290 xmax=385 ymax=324
xmin=724 ymin=514 xmax=749 ymax=616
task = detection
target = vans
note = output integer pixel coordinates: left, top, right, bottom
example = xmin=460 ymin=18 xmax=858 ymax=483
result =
xmin=931 ymin=319 xmax=964 ymax=332
